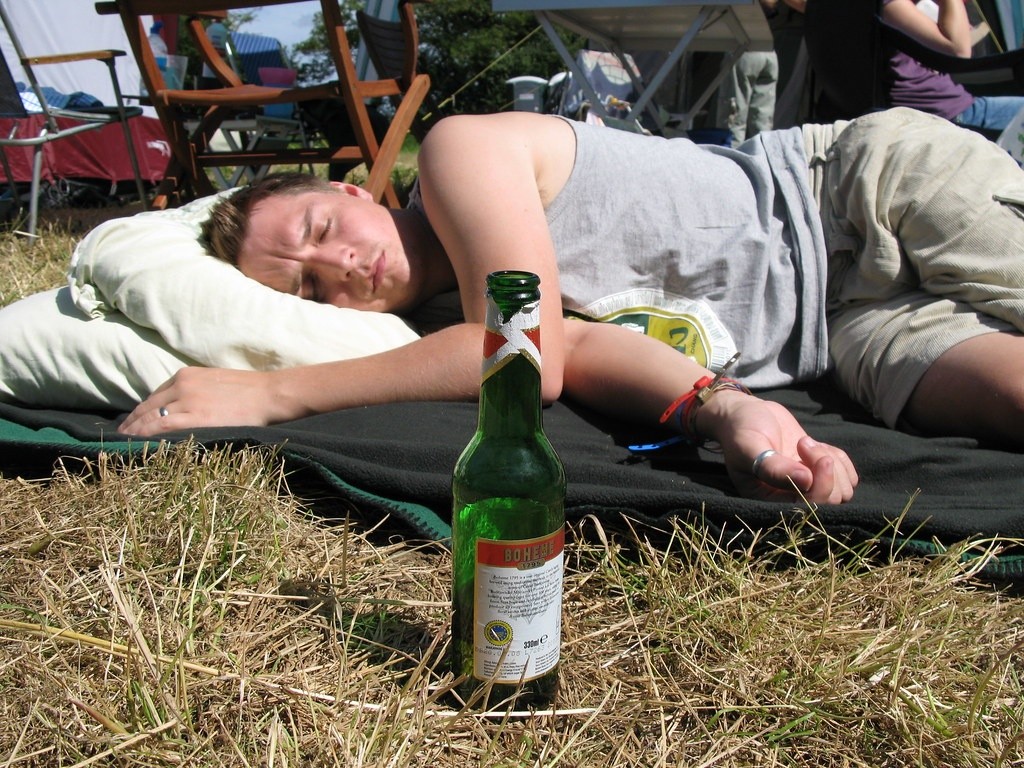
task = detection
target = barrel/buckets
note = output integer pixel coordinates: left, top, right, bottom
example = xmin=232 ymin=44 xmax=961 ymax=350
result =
xmin=685 ymin=128 xmax=733 ymax=148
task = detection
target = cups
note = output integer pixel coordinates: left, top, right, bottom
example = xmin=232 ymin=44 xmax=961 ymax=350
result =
xmin=168 ymin=55 xmax=188 ymax=91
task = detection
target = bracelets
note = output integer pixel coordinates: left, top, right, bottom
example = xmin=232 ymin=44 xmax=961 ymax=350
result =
xmin=658 ymin=353 xmax=755 ymax=456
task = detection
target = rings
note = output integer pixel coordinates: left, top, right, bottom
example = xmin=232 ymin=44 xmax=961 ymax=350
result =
xmin=753 ymin=448 xmax=776 ymax=475
xmin=160 ymin=407 xmax=168 ymax=417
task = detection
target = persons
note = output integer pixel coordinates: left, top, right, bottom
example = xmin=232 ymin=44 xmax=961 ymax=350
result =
xmin=763 ymin=1 xmax=1024 ymax=146
xmin=116 ymin=102 xmax=1024 ymax=504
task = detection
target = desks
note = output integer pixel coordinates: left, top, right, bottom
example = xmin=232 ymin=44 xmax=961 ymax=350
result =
xmin=492 ymin=0 xmax=774 ymax=138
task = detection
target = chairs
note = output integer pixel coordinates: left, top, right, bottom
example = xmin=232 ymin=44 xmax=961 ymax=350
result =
xmin=804 ymin=0 xmax=1024 ymax=142
xmin=96 ymin=0 xmax=432 ymax=209
xmin=0 ymin=0 xmax=148 ymax=247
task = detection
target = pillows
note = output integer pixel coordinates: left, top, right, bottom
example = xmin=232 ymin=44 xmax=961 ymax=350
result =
xmin=0 ymin=283 xmax=199 ymax=413
xmin=69 ymin=186 xmax=419 ymax=373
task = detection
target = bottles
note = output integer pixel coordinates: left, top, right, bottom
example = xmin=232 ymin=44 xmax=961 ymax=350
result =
xmin=139 ymin=20 xmax=169 ymax=97
xmin=201 ymin=18 xmax=227 ymax=89
xmin=449 ymin=271 xmax=568 ymax=720
